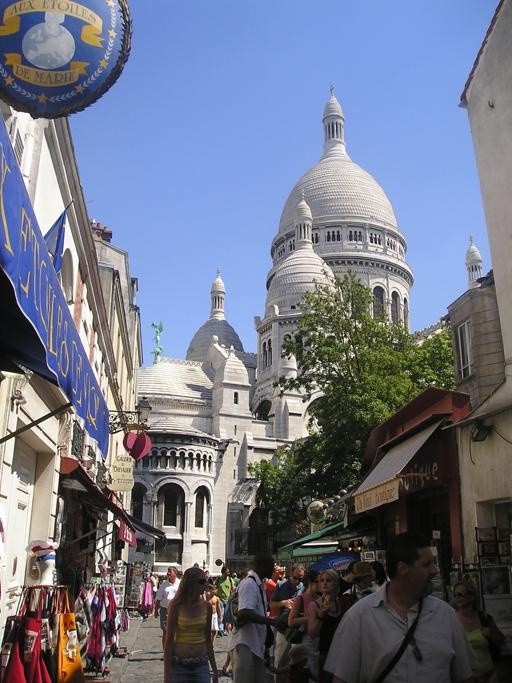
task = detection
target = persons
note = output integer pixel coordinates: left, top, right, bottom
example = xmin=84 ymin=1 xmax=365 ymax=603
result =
xmin=154 ymin=530 xmax=504 ymax=682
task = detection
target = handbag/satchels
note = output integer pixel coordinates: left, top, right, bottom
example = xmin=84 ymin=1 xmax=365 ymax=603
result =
xmin=277 ymin=612 xmax=306 ymax=644
xmin=1 ymin=607 xmax=84 ymax=683
xmin=223 ymin=593 xmax=248 ymax=630
xmin=265 ymin=625 xmax=274 ymax=649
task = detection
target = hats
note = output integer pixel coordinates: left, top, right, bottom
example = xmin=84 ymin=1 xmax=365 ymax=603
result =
xmin=288 ymin=643 xmax=313 ymax=666
xmin=348 ymin=562 xmax=376 ymax=584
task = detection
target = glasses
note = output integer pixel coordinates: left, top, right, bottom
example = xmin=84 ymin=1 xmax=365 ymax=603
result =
xmin=454 ymin=591 xmax=468 ymax=598
xmin=409 ymin=635 xmax=421 ymax=662
xmin=291 ymin=573 xmax=304 ymax=582
xmin=196 ymin=579 xmax=208 ymax=586
